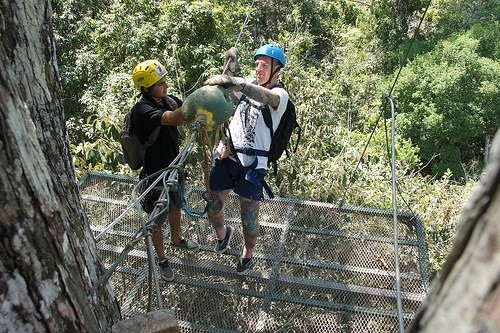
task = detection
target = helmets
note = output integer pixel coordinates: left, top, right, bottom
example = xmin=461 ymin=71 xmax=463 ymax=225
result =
xmin=133 ymin=60 xmax=168 ymax=91
xmin=254 ymin=44 xmax=285 ymax=68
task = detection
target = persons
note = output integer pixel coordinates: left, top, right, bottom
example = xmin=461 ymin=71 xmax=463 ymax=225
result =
xmin=130 ymin=59 xmax=202 ymax=283
xmin=203 ymin=43 xmax=288 ymax=274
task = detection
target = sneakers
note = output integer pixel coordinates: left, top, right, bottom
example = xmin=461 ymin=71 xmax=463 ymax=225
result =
xmin=214 ymin=225 xmax=233 ymax=254
xmin=156 ymin=257 xmax=174 ymax=281
xmin=236 ymin=244 xmax=253 ymax=275
xmin=170 ymin=236 xmax=200 ymax=251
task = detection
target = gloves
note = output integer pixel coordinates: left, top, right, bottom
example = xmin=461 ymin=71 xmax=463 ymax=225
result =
xmin=203 ymin=75 xmax=244 ymax=92
xmin=223 ymin=47 xmax=239 ymax=76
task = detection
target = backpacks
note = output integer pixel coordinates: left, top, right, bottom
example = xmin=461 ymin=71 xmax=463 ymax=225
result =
xmin=120 ymin=96 xmax=178 ymax=170
xmin=235 ymin=79 xmax=302 ymax=176
